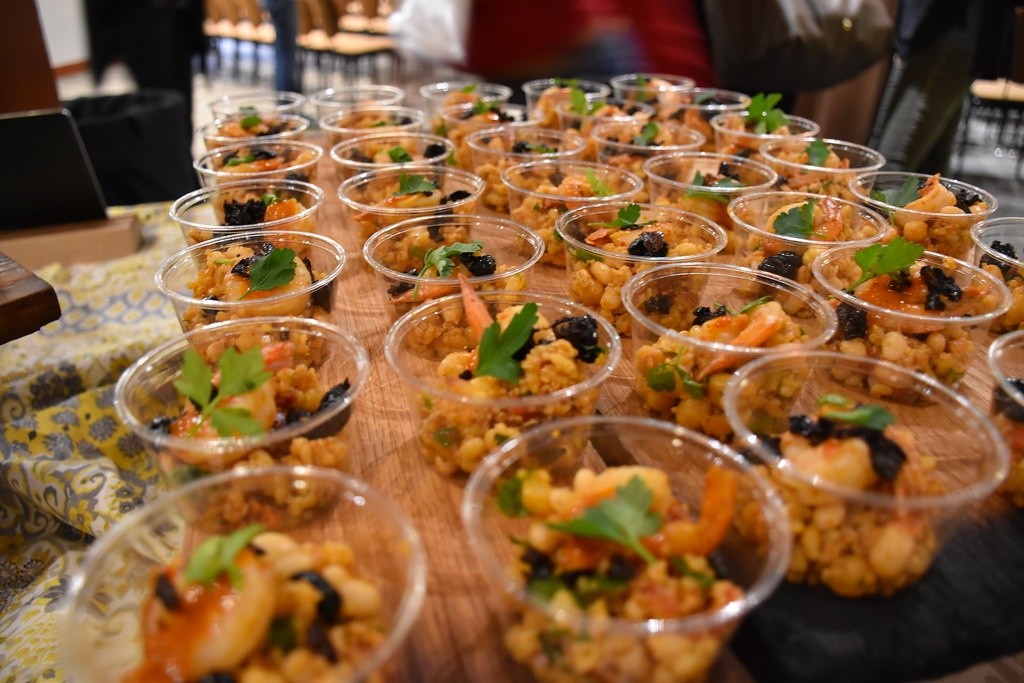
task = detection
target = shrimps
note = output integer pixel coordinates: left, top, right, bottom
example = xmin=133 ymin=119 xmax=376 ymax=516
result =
xmin=163 ymin=78 xmax=1024 ymax=619
xmin=134 ymin=526 xmax=287 ymax=677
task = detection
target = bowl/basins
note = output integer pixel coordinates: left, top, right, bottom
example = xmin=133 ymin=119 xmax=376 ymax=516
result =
xmin=621 ymin=262 xmax=838 ymax=442
xmin=462 ymin=418 xmax=791 ymax=683
xmin=115 ymin=316 xmax=369 ymax=491
xmin=724 ymin=351 xmax=1008 ymax=597
xmin=987 ymin=331 xmax=1024 ymax=511
xmin=155 ymin=70 xmax=1023 ymax=390
xmin=384 ymin=291 xmax=621 ymax=483
xmin=50 ymin=467 xmax=426 ymax=683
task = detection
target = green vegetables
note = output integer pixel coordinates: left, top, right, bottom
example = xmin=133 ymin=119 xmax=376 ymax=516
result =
xmin=171 ymin=70 xmax=925 ymax=608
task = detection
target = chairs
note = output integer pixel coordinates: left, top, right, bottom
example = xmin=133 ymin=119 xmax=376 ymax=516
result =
xmin=958 ymin=78 xmax=1024 ymax=180
xmin=201 ymin=0 xmax=408 ymax=90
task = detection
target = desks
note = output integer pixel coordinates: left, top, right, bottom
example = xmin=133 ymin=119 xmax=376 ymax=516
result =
xmin=184 ymin=130 xmax=1024 ymax=683
